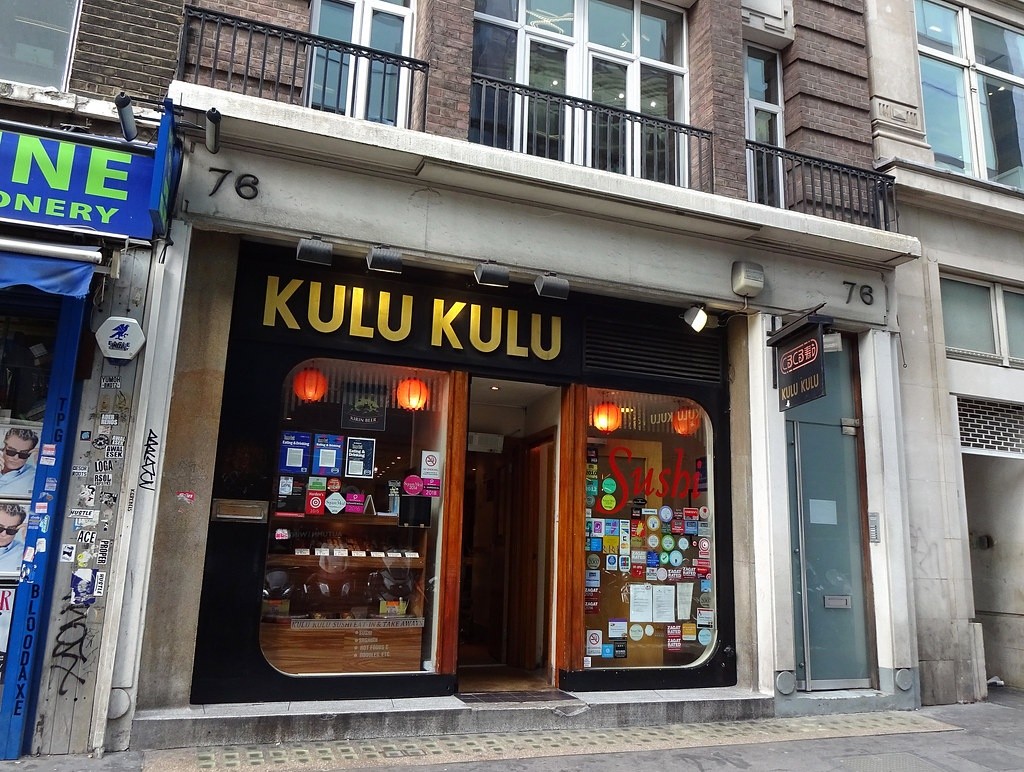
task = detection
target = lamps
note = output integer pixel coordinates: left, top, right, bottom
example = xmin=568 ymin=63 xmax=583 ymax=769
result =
xmin=678 ymin=302 xmax=708 ymax=333
xmin=365 ymin=244 xmax=402 ymax=274
xmin=473 ymin=260 xmax=509 ymax=288
xmin=533 ymin=271 xmax=569 ymax=300
xmin=296 ymin=235 xmax=333 ymax=265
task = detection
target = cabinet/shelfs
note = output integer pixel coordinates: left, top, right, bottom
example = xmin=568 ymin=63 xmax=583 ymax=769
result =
xmin=259 ymin=513 xmax=428 ymax=672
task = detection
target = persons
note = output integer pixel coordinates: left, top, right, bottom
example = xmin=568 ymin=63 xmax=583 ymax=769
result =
xmin=0 ymin=502 xmax=27 ymax=572
xmin=0 ymin=427 xmax=40 ymax=495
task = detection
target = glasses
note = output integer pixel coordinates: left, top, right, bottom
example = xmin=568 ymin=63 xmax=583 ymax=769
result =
xmin=0 ymin=527 xmax=19 ymax=535
xmin=5 ymin=448 xmax=31 ymax=459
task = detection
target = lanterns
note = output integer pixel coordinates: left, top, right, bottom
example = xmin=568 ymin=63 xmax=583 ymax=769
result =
xmin=671 ymin=406 xmax=701 ymax=435
xmin=589 ymin=402 xmax=622 ymax=435
xmin=397 ymin=377 xmax=427 ymax=411
xmin=293 ymin=367 xmax=328 ymax=403
